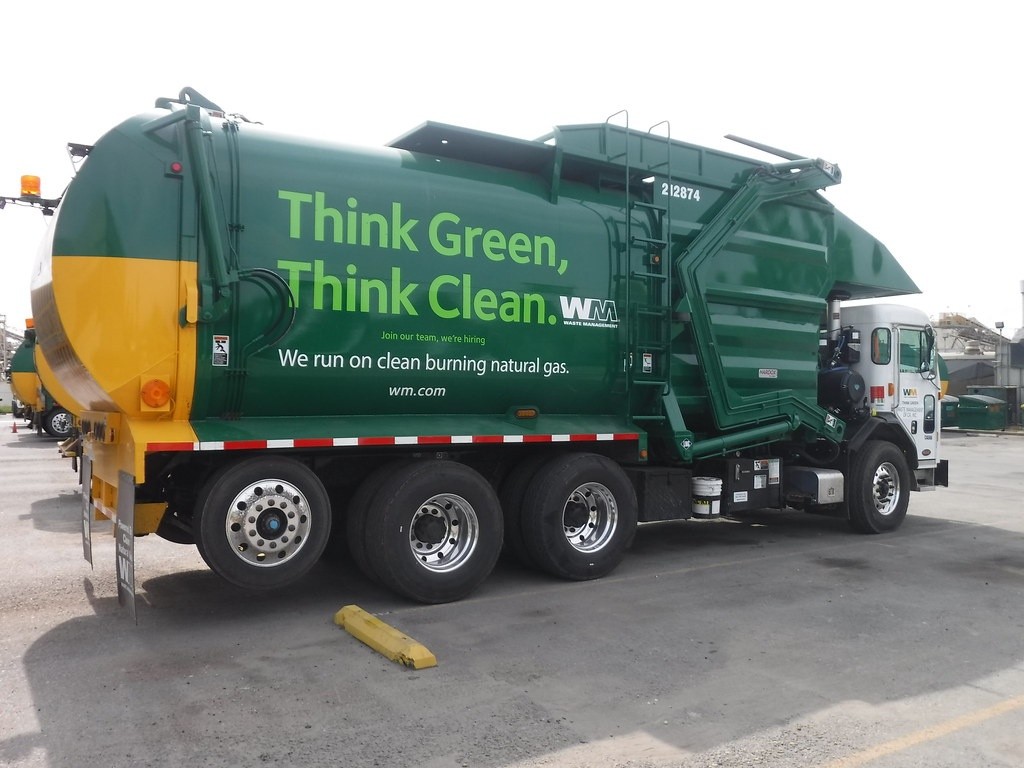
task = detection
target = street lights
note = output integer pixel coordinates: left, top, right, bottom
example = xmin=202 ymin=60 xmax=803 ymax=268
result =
xmin=996 ymin=321 xmax=1005 ymax=387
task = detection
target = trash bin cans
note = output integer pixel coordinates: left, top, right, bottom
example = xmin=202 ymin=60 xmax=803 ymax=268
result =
xmin=958 ymin=394 xmax=1007 ymax=430
xmin=941 ymin=395 xmax=959 ymax=428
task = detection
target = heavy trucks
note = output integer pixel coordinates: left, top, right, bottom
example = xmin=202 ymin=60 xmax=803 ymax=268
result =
xmin=1 ymin=87 xmax=951 ymax=627
